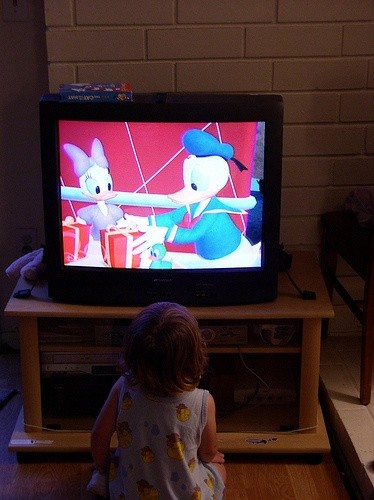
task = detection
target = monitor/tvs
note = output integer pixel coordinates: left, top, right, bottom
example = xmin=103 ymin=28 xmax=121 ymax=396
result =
xmin=38 ymin=92 xmax=283 ymax=306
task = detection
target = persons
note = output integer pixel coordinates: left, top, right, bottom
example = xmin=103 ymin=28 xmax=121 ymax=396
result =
xmin=85 ymin=301 xmax=228 ymax=500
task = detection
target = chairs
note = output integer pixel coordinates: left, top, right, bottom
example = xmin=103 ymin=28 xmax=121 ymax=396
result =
xmin=318 ymin=199 xmax=373 ymax=406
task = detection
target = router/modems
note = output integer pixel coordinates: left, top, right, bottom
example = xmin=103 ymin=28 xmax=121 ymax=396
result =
xmin=233 ymin=358 xmax=296 ymax=404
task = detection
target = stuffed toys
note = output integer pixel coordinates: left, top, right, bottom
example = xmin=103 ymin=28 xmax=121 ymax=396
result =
xmin=5 ymin=242 xmax=46 ymax=281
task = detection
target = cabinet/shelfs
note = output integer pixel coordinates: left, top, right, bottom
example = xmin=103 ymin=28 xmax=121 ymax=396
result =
xmin=2 ymin=239 xmax=333 ymax=455
